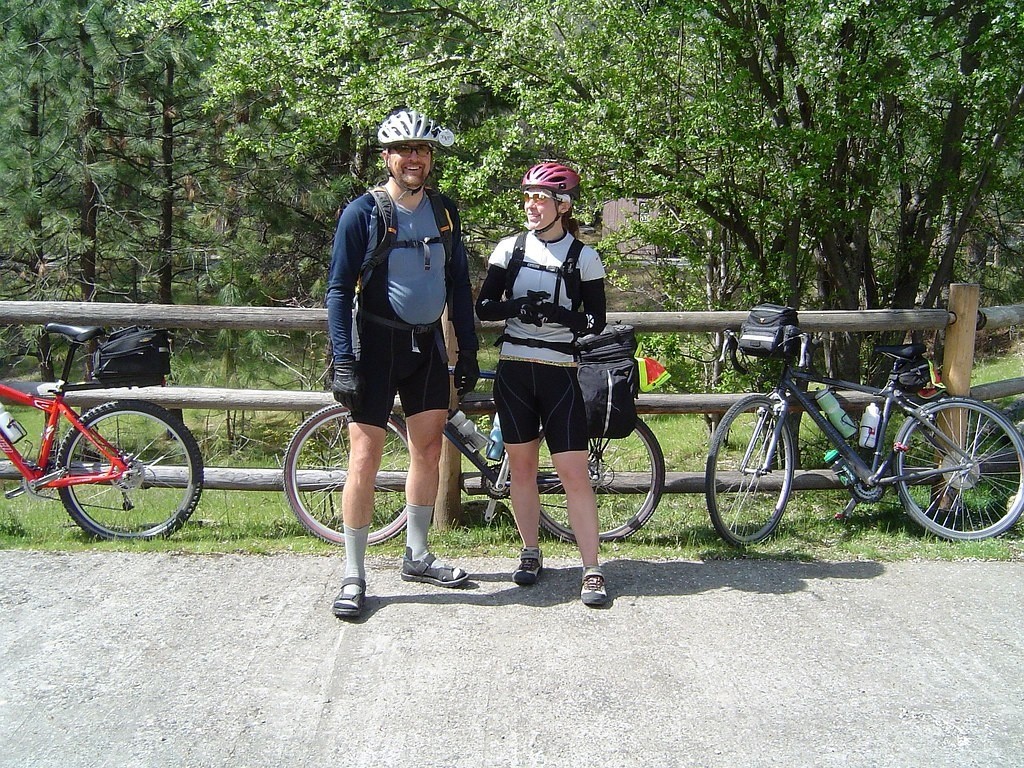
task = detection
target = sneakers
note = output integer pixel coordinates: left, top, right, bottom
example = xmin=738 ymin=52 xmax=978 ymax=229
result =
xmin=511 ymin=547 xmax=543 ymax=585
xmin=581 ymin=565 xmax=608 ymax=605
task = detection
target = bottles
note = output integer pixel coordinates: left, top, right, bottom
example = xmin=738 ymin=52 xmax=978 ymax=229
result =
xmin=486 ymin=411 xmax=504 ymax=460
xmin=822 ymin=449 xmax=856 ymax=486
xmin=0 ymin=401 xmax=24 ymax=443
xmin=858 ymin=402 xmax=881 ymax=449
xmin=446 ymin=408 xmax=488 ymax=450
xmin=814 ymin=386 xmax=857 ymax=439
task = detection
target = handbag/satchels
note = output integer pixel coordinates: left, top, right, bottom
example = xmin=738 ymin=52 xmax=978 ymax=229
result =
xmin=91 ymin=325 xmax=172 ymax=385
xmin=573 ymin=322 xmax=637 ymax=439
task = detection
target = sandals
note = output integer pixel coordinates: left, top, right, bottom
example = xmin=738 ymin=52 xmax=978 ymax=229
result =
xmin=333 ymin=577 xmax=366 ymax=618
xmin=401 ymin=546 xmax=469 ymax=587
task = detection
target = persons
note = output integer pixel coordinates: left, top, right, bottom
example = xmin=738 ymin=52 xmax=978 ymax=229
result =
xmin=475 ymin=162 xmax=608 ymax=604
xmin=324 ymin=108 xmax=480 ymax=616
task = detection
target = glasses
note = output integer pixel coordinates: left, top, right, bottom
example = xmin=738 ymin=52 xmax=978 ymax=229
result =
xmin=524 ymin=191 xmax=566 ymax=203
xmin=385 ymin=144 xmax=434 ymax=158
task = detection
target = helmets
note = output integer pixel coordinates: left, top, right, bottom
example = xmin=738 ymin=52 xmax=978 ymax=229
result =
xmin=378 ymin=111 xmax=439 ymax=145
xmin=522 ymin=163 xmax=581 ymax=194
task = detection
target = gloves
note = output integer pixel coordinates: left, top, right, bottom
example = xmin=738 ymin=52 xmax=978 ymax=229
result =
xmin=332 ymin=364 xmax=362 ymax=412
xmin=512 ymin=289 xmax=552 ymax=328
xmin=535 ymin=300 xmax=560 ymax=324
xmin=453 ymin=348 xmax=480 ymax=396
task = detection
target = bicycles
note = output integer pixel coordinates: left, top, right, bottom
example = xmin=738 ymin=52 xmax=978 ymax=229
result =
xmin=283 ymin=366 xmax=667 ymax=548
xmin=705 ymin=325 xmax=1024 ymax=549
xmin=1 ymin=322 xmax=204 ymax=541
